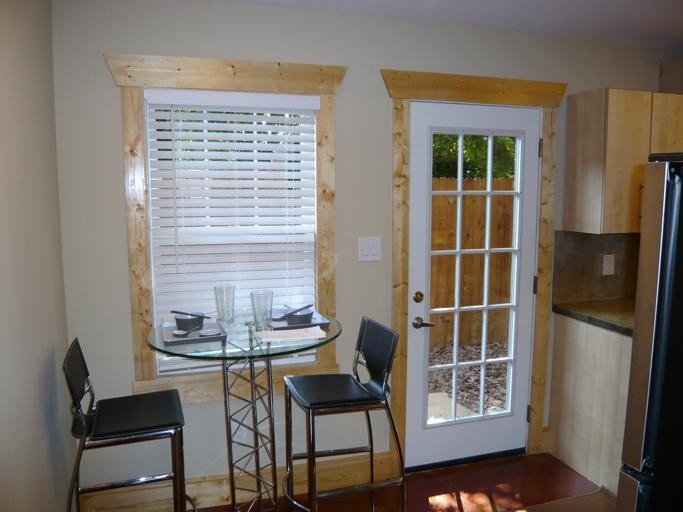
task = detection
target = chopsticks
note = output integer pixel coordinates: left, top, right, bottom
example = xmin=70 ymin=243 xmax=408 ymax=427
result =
xmin=281 ymin=304 xmax=313 ymax=320
xmin=171 ymin=311 xmax=211 ymax=319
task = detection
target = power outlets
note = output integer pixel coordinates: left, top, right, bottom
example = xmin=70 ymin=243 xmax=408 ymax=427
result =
xmin=357 ymin=236 xmax=382 ymax=263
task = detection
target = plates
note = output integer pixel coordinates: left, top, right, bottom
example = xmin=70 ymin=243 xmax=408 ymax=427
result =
xmin=160 ymin=318 xmax=228 ymax=346
xmin=264 ymin=308 xmax=329 ymax=328
xmin=199 ymin=329 xmax=219 ymax=335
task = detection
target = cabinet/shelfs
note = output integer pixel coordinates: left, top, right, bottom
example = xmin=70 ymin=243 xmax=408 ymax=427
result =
xmin=562 ymin=86 xmax=682 ymax=235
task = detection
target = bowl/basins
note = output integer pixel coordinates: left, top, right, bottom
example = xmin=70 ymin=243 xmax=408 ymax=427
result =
xmin=287 ymin=310 xmax=312 ymax=326
xmin=175 ymin=313 xmax=204 ymax=331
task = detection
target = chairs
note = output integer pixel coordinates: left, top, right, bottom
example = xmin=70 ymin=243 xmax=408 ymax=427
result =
xmin=282 ymin=315 xmax=408 ymax=512
xmin=61 ymin=336 xmax=186 ymax=512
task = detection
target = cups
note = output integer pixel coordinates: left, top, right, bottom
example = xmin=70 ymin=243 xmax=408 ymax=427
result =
xmin=251 ymin=290 xmax=273 ymax=331
xmin=214 ymin=285 xmax=235 ymax=322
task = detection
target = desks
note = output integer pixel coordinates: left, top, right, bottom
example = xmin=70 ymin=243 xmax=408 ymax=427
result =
xmin=549 ymin=297 xmax=637 ymax=496
xmin=145 ymin=309 xmax=343 ymax=512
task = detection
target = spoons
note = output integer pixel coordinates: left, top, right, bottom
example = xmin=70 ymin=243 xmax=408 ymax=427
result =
xmin=173 ymin=325 xmax=201 ymax=337
